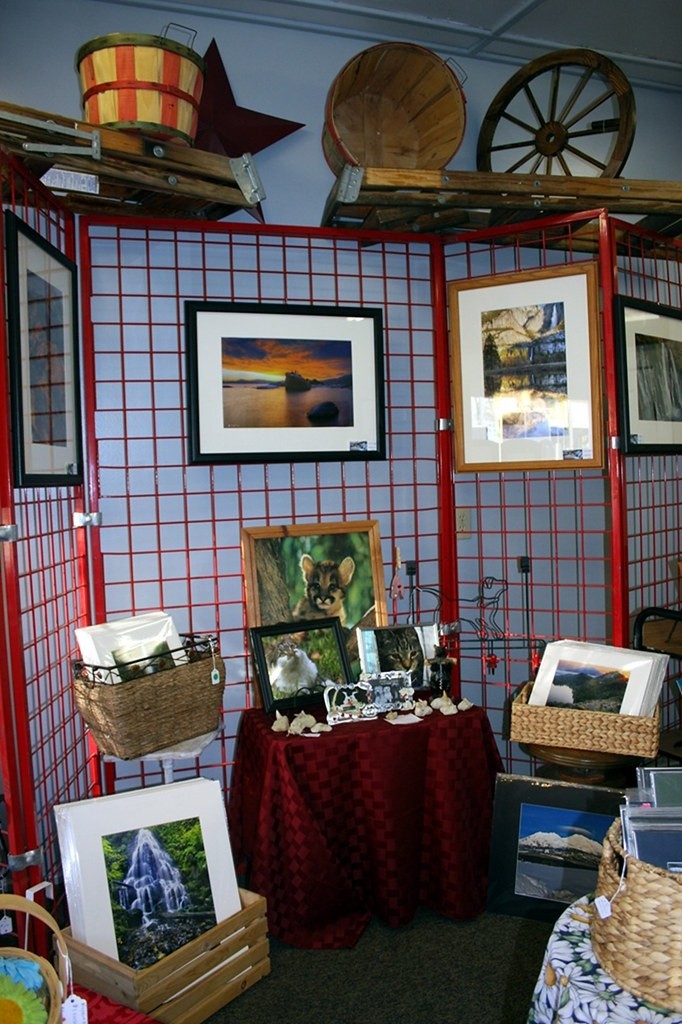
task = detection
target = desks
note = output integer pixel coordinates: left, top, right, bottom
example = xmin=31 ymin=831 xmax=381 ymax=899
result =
xmin=230 ymin=698 xmax=505 ymax=949
xmin=529 ymin=893 xmax=682 ymax=1024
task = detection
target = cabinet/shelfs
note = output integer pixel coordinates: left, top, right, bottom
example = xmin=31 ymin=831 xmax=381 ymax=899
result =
xmin=632 ymin=606 xmax=682 ymax=786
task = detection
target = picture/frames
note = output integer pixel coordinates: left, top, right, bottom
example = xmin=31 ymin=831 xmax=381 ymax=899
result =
xmin=247 ymin=615 xmax=352 ymax=716
xmin=526 ymin=639 xmax=670 ymax=719
xmin=612 ymin=293 xmax=682 ymax=459
xmin=360 ymin=670 xmax=414 ymax=712
xmin=357 ymin=622 xmax=444 ymax=692
xmin=239 ymin=516 xmax=389 ymax=709
xmin=53 ymin=774 xmax=252 ymax=1002
xmin=482 ymin=771 xmax=626 ymax=925
xmin=445 ymin=261 xmax=607 ymax=470
xmin=182 ymin=299 xmax=387 ymax=465
xmin=4 ymin=207 xmax=85 ymax=491
xmin=74 ymin=610 xmax=192 ymax=684
xmin=324 ymin=681 xmax=378 ymax=725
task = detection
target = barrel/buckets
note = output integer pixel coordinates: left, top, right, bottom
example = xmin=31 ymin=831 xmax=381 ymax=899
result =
xmin=74 ymin=22 xmax=206 ymax=148
xmin=321 ymin=41 xmax=468 ymax=178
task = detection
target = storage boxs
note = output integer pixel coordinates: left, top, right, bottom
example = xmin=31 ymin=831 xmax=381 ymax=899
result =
xmin=52 ymin=882 xmax=273 ymax=1024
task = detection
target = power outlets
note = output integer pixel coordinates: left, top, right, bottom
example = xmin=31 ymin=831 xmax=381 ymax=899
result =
xmin=456 ymin=508 xmax=472 ymax=540
